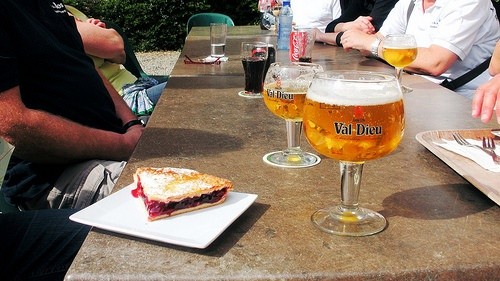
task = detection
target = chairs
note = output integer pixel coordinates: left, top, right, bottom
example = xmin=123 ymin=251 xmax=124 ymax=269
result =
xmin=187 ymin=13 xmax=235 ymax=34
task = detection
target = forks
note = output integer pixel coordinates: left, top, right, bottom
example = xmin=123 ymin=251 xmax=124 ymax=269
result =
xmin=451 ymin=133 xmax=500 ymax=165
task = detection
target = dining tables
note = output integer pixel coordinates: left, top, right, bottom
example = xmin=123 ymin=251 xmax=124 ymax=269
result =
xmin=65 ymin=26 xmax=500 ymax=281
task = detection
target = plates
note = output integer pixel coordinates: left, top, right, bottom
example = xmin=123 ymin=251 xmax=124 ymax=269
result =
xmin=68 ymin=183 xmax=258 ymax=249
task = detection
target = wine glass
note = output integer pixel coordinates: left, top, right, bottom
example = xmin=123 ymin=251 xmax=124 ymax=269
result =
xmin=303 ymin=70 xmax=405 ymax=237
xmin=262 ymin=62 xmax=324 ymax=168
xmin=382 ymin=34 xmax=418 ymax=92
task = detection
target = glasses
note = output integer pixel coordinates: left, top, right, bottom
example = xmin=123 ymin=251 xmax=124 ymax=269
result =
xmin=182 ymin=54 xmax=223 ymax=65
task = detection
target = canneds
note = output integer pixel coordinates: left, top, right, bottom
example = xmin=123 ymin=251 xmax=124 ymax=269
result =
xmin=289 ymin=30 xmax=308 ymax=62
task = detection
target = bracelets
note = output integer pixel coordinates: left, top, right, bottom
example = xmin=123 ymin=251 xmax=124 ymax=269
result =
xmin=371 ymin=37 xmax=381 ymax=57
xmin=120 ymin=119 xmax=146 ymax=133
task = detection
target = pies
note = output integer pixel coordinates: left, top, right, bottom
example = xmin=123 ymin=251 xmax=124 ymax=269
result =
xmin=131 ymin=167 xmax=231 ymax=219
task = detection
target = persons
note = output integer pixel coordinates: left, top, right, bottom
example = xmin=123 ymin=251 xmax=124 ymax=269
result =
xmin=0 ymin=0 xmax=147 ymax=210
xmin=306 ymin=0 xmax=399 ymax=48
xmin=290 ymin=0 xmax=342 ymax=34
xmin=471 ymin=39 xmax=500 ymax=125
xmin=0 ymin=209 xmax=92 ymax=281
xmin=339 ymin=0 xmax=500 ymax=100
xmin=65 ymin=5 xmax=170 ymax=124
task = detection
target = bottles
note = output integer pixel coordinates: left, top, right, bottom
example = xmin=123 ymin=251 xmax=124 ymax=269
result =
xmin=276 ymin=0 xmax=293 ymax=49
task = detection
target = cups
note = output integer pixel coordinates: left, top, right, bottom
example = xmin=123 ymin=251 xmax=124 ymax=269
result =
xmin=297 ymin=28 xmax=317 ymax=63
xmin=210 ymin=23 xmax=227 ymax=57
xmin=241 ymin=41 xmax=275 ymax=94
